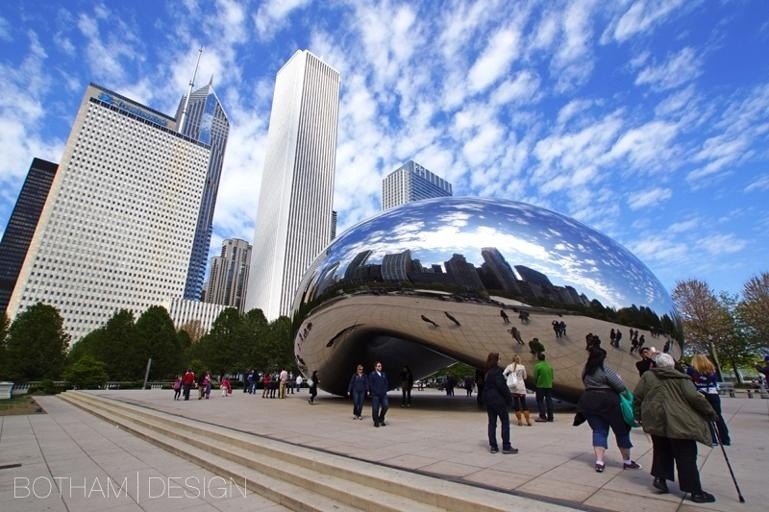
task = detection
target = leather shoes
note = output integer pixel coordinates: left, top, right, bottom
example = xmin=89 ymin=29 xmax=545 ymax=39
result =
xmin=652 ymin=476 xmax=670 ymax=494
xmin=690 ymin=492 xmax=717 ymax=505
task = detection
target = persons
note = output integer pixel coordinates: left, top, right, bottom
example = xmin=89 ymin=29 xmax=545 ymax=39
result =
xmin=442 ymin=310 xmax=461 ymax=327
xmin=420 ymin=314 xmax=441 ymax=328
xmin=347 ymin=362 xmax=370 ymax=422
xmin=533 ymin=351 xmax=554 ymax=423
xmin=294 ymin=321 xmax=313 ymax=376
xmin=306 ymin=369 xmax=321 ymax=406
xmin=499 ymin=308 xmax=511 ymax=325
xmin=399 ymin=364 xmax=414 ymax=406
xmin=416 ymin=369 xmax=486 ymax=411
xmin=366 ymin=359 xmax=391 ymax=428
xmin=517 ymin=309 xmax=670 ymax=355
xmin=502 ymin=354 xmax=532 ymax=426
xmin=633 ymin=347 xmax=768 ymax=503
xmin=575 ymin=346 xmax=642 ymax=473
xmin=485 ymin=352 xmax=519 ymax=455
xmin=506 ymin=326 xmax=526 ymax=346
xmin=528 ymin=337 xmax=546 ymax=361
xmin=171 ymin=367 xmax=304 ymax=401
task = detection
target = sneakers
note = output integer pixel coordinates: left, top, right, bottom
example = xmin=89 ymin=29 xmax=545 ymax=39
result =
xmin=501 ymin=447 xmax=519 ymax=455
xmin=517 ymin=417 xmax=554 ymax=426
xmin=622 ymin=461 xmax=642 ymax=471
xmin=352 ymin=414 xmax=363 ymax=420
xmin=594 ymin=462 xmax=607 ymax=472
xmin=373 ymin=420 xmax=386 ymax=427
xmin=490 ymin=448 xmax=498 ymax=454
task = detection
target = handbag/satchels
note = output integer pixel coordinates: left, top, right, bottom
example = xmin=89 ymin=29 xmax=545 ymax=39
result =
xmin=618 ymin=384 xmax=643 ymax=427
